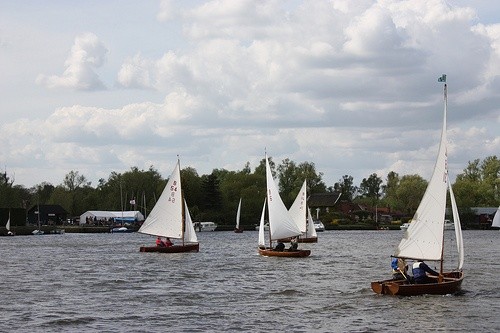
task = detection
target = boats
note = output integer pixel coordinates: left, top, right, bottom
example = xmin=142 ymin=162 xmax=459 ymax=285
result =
xmin=110 ymin=226 xmax=133 ymax=233
xmin=255 ymin=222 xmax=270 ymax=231
xmin=307 ymin=219 xmax=326 ymax=232
xmin=193 ymin=221 xmax=218 ymax=232
xmin=399 ymin=218 xmax=457 ymax=231
xmin=49 ymin=228 xmax=66 ymax=234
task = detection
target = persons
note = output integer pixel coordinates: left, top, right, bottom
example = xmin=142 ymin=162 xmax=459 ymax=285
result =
xmin=164 ymin=237 xmax=174 ymax=247
xmin=412 ymin=259 xmax=440 ymax=284
xmin=156 ymin=236 xmax=166 ymax=247
xmin=391 ymin=258 xmax=410 ymax=281
xmin=86 ymin=216 xmax=114 ymax=226
xmin=288 ymin=237 xmax=298 ymax=252
xmin=272 ymin=240 xmax=285 ymax=252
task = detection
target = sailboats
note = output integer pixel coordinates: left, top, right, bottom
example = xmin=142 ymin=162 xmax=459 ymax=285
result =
xmin=234 ymin=198 xmax=244 ymax=233
xmin=6 ymin=210 xmax=17 ymax=236
xmin=370 ymin=72 xmax=465 ymax=297
xmin=256 ymin=146 xmax=311 ymax=258
xmin=136 ymin=153 xmax=200 ymax=253
xmin=277 ymin=196 xmax=318 ymax=244
xmin=31 ymin=204 xmax=45 ymax=235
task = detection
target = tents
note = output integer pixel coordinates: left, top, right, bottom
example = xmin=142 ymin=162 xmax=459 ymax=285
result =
xmin=79 ymin=211 xmax=144 ymax=225
